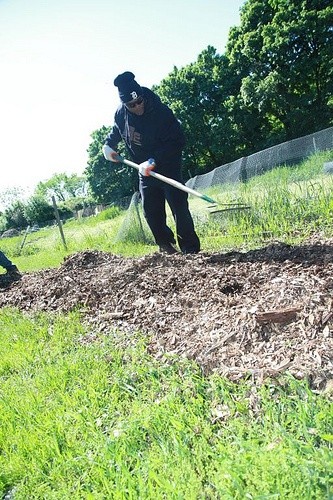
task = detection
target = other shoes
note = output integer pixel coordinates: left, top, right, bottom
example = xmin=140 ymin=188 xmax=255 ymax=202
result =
xmin=7 ymin=265 xmax=18 ymax=271
xmin=159 ymin=243 xmax=178 ymax=255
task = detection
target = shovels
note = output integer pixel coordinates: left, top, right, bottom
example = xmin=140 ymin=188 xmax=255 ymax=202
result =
xmin=112 ymin=154 xmax=251 ymax=215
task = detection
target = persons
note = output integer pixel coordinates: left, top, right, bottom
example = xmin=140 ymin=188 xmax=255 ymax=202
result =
xmin=102 ymin=71 xmax=201 ymax=256
xmin=0 ymin=250 xmax=23 ymax=289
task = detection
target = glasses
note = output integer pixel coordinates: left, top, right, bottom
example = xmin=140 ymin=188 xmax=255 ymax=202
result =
xmin=123 ymin=95 xmax=143 ymax=108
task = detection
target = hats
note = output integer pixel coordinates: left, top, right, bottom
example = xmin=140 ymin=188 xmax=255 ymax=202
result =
xmin=114 ymin=71 xmax=143 ymax=103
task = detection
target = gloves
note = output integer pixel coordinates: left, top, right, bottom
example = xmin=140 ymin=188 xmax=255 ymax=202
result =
xmin=139 ymin=158 xmax=156 ymax=177
xmin=102 ymin=145 xmax=118 ymax=162
